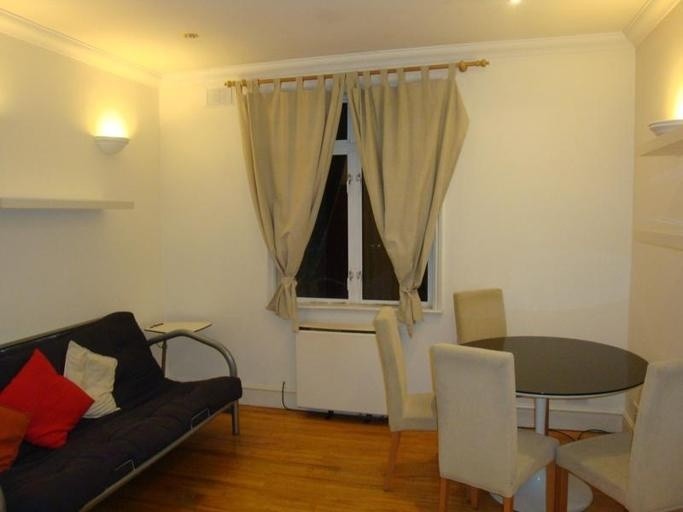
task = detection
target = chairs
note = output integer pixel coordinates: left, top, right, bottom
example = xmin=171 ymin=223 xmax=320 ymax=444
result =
xmin=453 ymin=287 xmax=508 ymax=343
xmin=555 ymin=358 xmax=681 ymax=511
xmin=428 ymin=342 xmax=558 ymax=510
xmin=375 ymin=305 xmax=439 ymax=493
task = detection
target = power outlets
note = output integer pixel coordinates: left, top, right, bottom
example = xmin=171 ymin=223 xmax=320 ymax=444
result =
xmin=279 ymin=375 xmax=288 ymax=389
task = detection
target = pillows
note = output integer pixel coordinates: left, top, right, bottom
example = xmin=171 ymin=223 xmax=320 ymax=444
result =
xmin=0 ymin=339 xmax=121 ymax=473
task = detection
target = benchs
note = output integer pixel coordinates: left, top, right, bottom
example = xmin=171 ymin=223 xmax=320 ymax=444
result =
xmin=0 ymin=310 xmax=243 ymax=510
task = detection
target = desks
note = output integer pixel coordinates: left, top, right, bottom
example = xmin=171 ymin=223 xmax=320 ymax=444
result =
xmin=146 ymin=321 xmax=213 ymax=377
